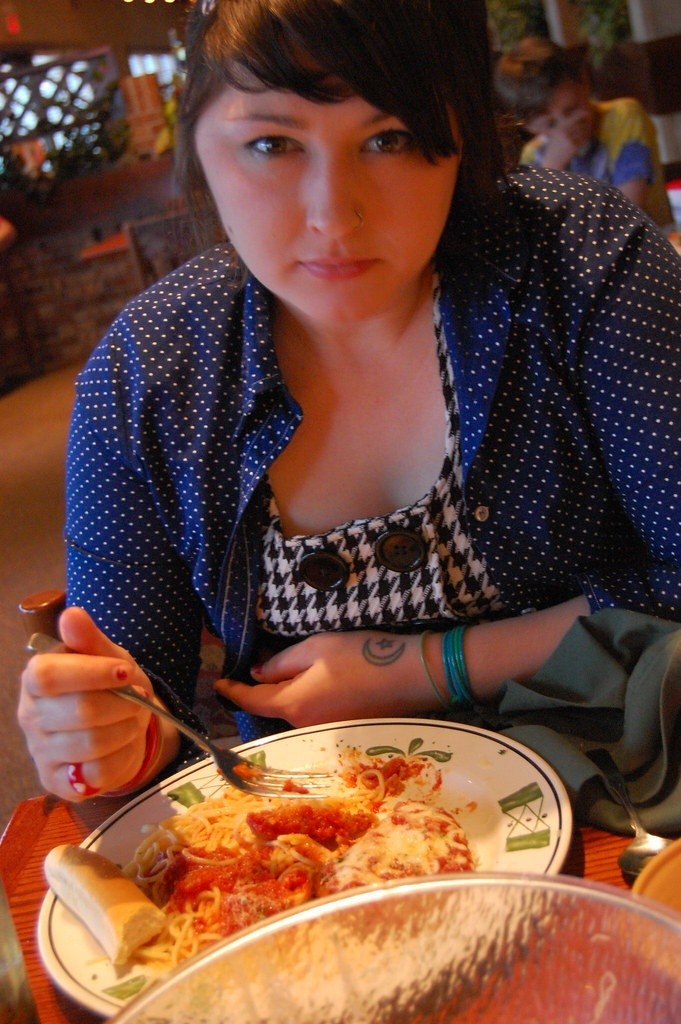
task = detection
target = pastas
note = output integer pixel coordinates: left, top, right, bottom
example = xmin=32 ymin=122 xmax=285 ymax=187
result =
xmin=120 ymin=771 xmax=475 ymax=979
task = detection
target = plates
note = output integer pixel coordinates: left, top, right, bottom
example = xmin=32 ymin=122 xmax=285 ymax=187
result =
xmin=35 ymin=717 xmax=574 ymax=1020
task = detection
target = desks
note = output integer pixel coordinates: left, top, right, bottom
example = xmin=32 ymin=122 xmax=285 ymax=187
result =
xmin=0 ymin=773 xmax=681 ymax=1024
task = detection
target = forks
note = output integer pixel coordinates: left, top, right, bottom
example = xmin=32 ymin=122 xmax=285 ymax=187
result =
xmin=27 ymin=631 xmax=332 ymax=800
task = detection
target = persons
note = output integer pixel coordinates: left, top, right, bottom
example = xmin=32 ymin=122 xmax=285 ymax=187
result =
xmin=494 ymin=34 xmax=675 ymax=234
xmin=18 ymin=1 xmax=681 ymax=807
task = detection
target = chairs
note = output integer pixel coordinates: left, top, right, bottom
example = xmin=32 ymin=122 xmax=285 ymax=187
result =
xmin=123 ymin=202 xmax=214 ymax=293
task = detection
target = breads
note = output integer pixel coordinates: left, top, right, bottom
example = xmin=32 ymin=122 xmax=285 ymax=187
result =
xmin=44 ymin=843 xmax=164 ymax=967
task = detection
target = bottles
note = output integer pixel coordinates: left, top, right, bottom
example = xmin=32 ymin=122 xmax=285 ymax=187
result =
xmin=0 ymin=878 xmax=40 ymax=1024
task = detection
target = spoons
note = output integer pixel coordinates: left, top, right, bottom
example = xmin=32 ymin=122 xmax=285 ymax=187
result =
xmin=588 ymin=748 xmax=674 ymax=875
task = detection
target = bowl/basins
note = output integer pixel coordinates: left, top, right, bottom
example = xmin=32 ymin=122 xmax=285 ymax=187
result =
xmin=104 ymin=871 xmax=680 ymax=1024
xmin=631 ymin=838 xmax=681 ymax=923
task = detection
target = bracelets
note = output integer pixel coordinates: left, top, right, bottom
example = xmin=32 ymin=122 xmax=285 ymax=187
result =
xmin=420 ymin=623 xmax=478 ymax=710
xmin=99 ymin=712 xmax=163 ymax=797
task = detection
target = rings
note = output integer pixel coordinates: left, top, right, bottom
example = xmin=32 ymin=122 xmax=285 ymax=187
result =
xmin=67 ymin=764 xmax=100 ymax=800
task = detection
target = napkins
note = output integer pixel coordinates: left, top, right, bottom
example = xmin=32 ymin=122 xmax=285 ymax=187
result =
xmin=486 ymin=604 xmax=681 ymax=836
